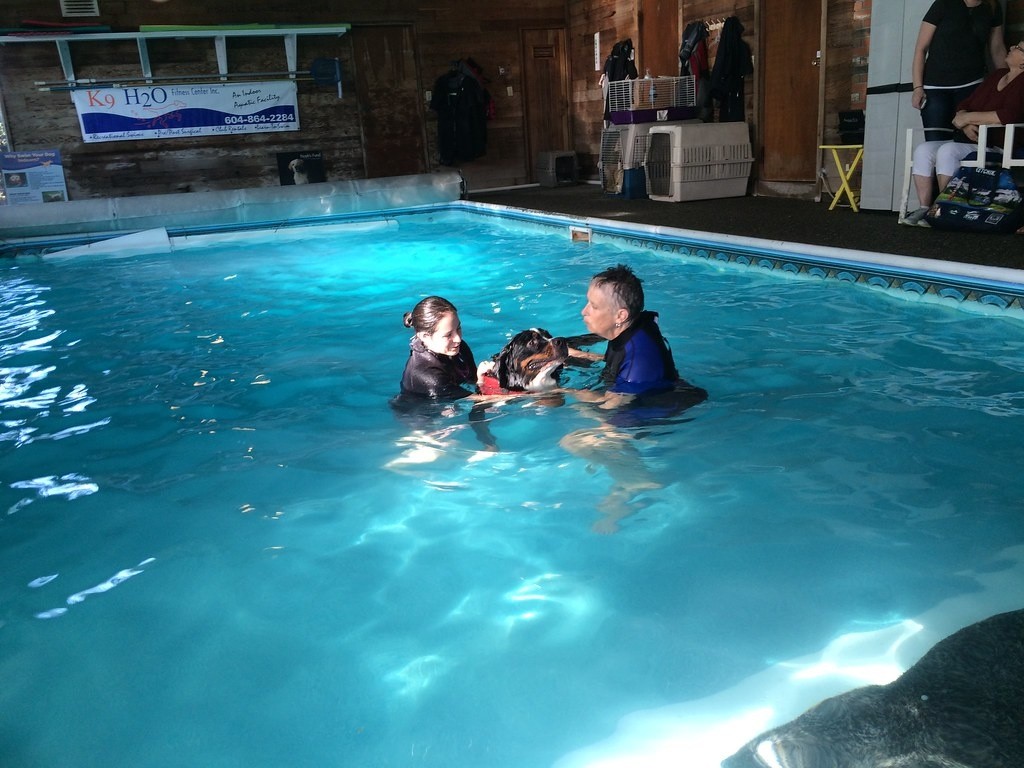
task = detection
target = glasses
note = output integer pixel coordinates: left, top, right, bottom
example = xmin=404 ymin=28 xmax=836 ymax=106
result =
xmin=1015 ymin=44 xmax=1024 ymax=52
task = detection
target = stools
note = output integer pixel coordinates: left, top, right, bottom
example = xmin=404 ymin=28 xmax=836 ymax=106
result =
xmin=819 ymin=144 xmax=864 ymax=212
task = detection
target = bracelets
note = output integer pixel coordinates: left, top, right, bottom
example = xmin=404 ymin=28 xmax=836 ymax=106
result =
xmin=914 ymin=86 xmax=922 ymax=89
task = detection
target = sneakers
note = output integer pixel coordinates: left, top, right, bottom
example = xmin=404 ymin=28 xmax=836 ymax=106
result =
xmin=903 ymin=207 xmax=930 ymax=226
xmin=918 ymin=219 xmax=932 ymax=227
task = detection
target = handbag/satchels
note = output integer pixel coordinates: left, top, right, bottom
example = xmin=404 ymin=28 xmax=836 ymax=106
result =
xmin=925 ymin=166 xmax=1022 ymax=237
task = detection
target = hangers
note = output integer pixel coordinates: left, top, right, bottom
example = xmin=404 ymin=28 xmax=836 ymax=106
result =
xmin=446 ymin=61 xmax=465 ymax=84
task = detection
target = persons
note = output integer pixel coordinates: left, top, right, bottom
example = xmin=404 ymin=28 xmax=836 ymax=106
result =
xmin=900 ymin=40 xmax=1024 ymax=227
xmin=568 ymin=264 xmax=679 ymax=381
xmin=912 ymin=0 xmax=1009 ymax=142
xmin=400 ymin=295 xmax=478 ymax=398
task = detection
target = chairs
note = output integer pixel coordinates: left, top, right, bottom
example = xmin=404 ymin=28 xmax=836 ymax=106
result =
xmin=1001 ymin=123 xmax=1023 ymax=169
xmin=898 ymin=123 xmax=1006 ymax=225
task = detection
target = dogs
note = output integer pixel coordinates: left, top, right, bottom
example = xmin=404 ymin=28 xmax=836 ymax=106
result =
xmin=491 ymin=328 xmax=570 ymax=390
